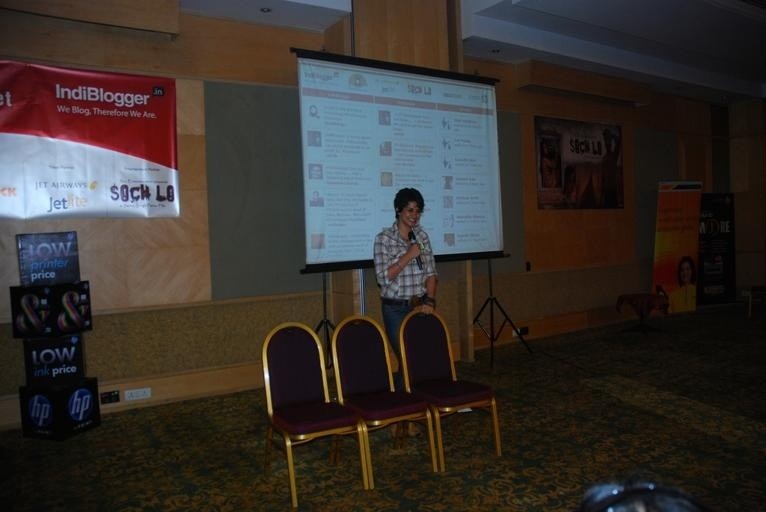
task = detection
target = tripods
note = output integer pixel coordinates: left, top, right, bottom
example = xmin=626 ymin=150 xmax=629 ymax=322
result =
xmin=314 ymin=273 xmax=338 ymax=372
xmin=473 ymin=256 xmax=532 ymax=364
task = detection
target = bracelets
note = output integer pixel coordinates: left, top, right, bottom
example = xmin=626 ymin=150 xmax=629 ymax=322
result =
xmin=395 ymin=262 xmax=404 ymax=271
xmin=424 ymin=296 xmax=436 ymax=308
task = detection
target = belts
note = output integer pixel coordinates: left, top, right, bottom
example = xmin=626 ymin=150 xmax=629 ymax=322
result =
xmin=380 ymin=291 xmax=429 ymax=307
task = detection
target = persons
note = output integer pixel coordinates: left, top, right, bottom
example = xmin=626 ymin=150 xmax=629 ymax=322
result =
xmin=667 ymin=256 xmax=697 ymax=315
xmin=372 ymin=186 xmax=439 ymax=442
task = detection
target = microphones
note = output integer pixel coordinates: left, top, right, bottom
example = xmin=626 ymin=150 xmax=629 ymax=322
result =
xmin=407 ymin=228 xmax=424 ymax=273
